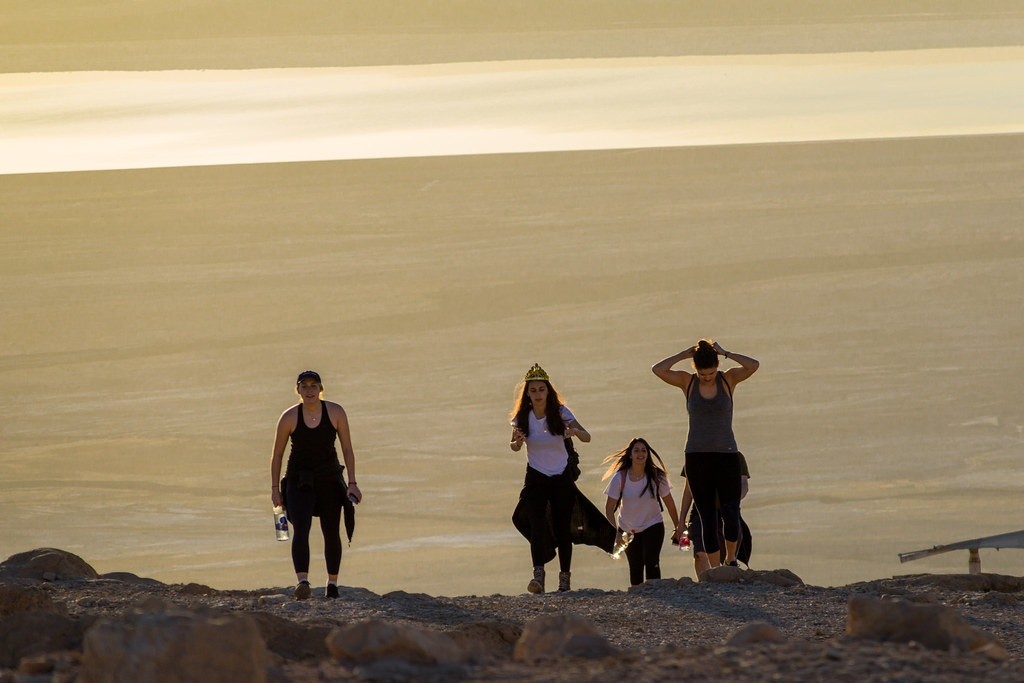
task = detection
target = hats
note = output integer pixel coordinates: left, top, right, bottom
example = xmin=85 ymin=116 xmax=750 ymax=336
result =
xmin=297 ymin=371 xmax=321 ymax=383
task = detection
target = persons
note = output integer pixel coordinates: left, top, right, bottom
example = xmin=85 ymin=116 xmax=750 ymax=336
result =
xmin=271 ymin=371 xmax=362 ymax=599
xmin=651 ymin=340 xmax=753 ymax=582
xmin=509 ymin=362 xmax=591 ymax=594
xmin=604 ymin=438 xmax=678 ymax=587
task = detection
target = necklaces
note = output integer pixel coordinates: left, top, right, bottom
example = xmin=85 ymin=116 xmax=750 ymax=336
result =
xmin=309 ymin=415 xmax=319 ymax=421
xmin=628 ymin=469 xmax=643 ymax=480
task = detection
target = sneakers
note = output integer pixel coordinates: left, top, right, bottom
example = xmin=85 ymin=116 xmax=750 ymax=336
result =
xmin=724 ymin=556 xmax=740 ymax=567
xmin=295 ymin=581 xmax=312 ymax=601
xmin=326 ymin=584 xmax=339 ymax=598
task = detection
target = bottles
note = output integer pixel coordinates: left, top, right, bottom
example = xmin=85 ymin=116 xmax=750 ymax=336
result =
xmin=274 ymin=505 xmax=289 ymax=541
xmin=679 ymin=521 xmax=692 ymax=552
xmin=608 ymin=529 xmax=635 ymax=560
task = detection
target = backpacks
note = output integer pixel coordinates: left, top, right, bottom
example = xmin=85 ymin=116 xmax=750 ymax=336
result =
xmin=563 ymin=427 xmax=580 ymax=465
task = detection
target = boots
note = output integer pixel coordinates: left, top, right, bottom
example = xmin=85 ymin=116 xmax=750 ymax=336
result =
xmin=527 ymin=568 xmax=545 ymax=594
xmin=557 ymin=572 xmax=571 ymax=592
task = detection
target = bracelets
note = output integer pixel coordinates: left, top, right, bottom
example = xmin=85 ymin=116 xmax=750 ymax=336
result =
xmin=516 ymin=442 xmax=521 ymax=447
xmin=272 ymin=486 xmax=279 ymax=488
xmin=724 ymin=352 xmax=731 ymax=359
xmin=348 ymin=482 xmax=357 ymax=485
